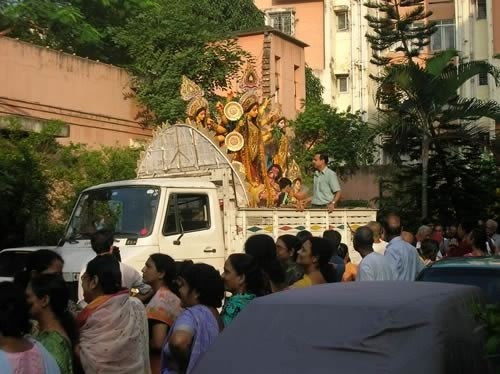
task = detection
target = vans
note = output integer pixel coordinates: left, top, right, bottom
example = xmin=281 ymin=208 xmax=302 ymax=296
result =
xmin=414 ymin=256 xmax=500 ymax=359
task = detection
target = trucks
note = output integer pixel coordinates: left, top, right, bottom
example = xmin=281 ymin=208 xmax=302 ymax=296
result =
xmin=0 ymin=170 xmax=381 ymax=306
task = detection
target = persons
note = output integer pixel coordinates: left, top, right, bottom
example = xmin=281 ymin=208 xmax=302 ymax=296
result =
xmin=77 ymin=229 xmax=154 ymax=303
xmin=402 ymin=216 xmax=500 ymax=265
xmin=14 ymin=249 xmax=81 ymax=346
xmin=220 ymin=234 xmax=286 ymax=328
xmin=353 ymin=226 xmax=392 ymax=281
xmin=305 ymin=150 xmax=341 ymax=212
xmin=383 ymin=214 xmax=424 ymax=281
xmin=26 ymin=273 xmax=73 ymax=374
xmin=73 ymin=254 xmax=153 ymax=374
xmin=323 ymin=230 xmax=357 ymax=282
xmin=276 ymin=234 xmax=304 ymax=287
xmin=142 ymin=253 xmax=224 ymax=374
xmin=297 ymin=230 xmax=312 ymax=241
xmin=277 ymin=178 xmax=300 ymax=208
xmin=0 ymin=281 xmax=61 ymax=374
xmin=286 ymin=237 xmax=337 ymax=289
xmin=367 ymin=221 xmax=390 ymax=255
xmin=187 ymin=90 xmax=306 ymax=209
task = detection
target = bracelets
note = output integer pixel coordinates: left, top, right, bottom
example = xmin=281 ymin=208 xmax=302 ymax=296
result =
xmin=332 ymin=201 xmax=335 ymax=204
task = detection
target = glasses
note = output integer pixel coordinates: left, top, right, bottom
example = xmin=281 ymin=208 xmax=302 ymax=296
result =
xmin=81 ymin=275 xmax=91 ymax=280
